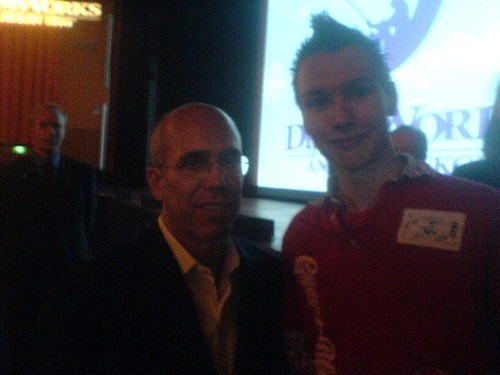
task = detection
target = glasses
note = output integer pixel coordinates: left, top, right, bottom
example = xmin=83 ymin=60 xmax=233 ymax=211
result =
xmin=162 ymin=153 xmax=251 ymax=178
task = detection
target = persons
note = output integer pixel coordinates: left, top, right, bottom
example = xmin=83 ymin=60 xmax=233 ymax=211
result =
xmin=14 ymin=102 xmax=282 ymax=375
xmin=282 ymin=11 xmax=500 ymax=375
xmin=0 ymin=103 xmax=97 ymax=288
